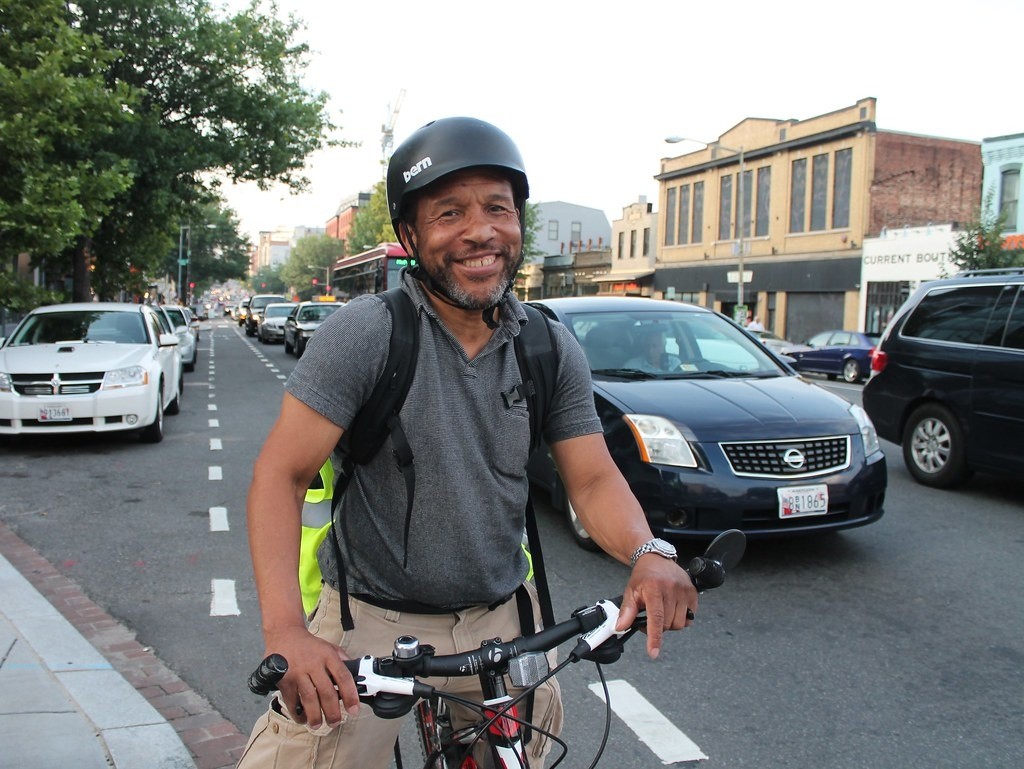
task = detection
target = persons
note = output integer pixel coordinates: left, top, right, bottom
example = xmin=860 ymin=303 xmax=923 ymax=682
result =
xmin=228 ymin=115 xmax=700 ymax=769
xmin=621 ymin=331 xmax=681 ymax=373
xmin=744 ymin=315 xmax=765 ymax=330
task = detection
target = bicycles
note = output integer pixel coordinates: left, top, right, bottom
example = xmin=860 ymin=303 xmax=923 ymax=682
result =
xmin=243 ymin=554 xmax=725 ymax=768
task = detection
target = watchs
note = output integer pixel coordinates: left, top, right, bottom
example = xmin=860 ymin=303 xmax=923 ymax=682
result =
xmin=629 ymin=538 xmax=678 ymax=566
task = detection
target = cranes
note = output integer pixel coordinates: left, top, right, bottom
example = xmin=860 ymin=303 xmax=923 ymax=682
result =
xmin=379 ymin=87 xmax=407 ymax=185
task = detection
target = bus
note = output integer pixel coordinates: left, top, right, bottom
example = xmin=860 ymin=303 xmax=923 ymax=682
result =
xmin=329 ymin=242 xmax=416 ymax=303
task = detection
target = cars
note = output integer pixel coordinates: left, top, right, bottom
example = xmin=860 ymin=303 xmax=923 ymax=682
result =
xmin=253 ymin=301 xmax=303 ymax=344
xmin=517 ymin=295 xmax=890 ymax=553
xmin=223 ymin=294 xmax=286 ymax=337
xmin=781 ymin=330 xmax=884 ymax=384
xmin=0 ymin=301 xmax=185 ymax=444
xmin=746 ymin=328 xmax=794 ymax=365
xmin=149 ymin=304 xmax=207 ymax=395
xmin=282 ymin=295 xmax=347 ymax=358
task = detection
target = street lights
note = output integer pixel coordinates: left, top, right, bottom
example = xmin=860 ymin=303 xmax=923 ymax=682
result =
xmin=307 ymin=265 xmax=329 ymax=296
xmin=178 ymin=224 xmax=216 ymax=298
xmin=665 ymin=135 xmax=745 ymax=327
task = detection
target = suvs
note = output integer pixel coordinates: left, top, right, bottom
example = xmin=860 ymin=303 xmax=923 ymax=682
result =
xmin=859 ymin=267 xmax=1023 ymax=490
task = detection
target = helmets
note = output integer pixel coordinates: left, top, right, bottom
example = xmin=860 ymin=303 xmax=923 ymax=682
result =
xmin=386 ymin=116 xmax=530 ymax=254
